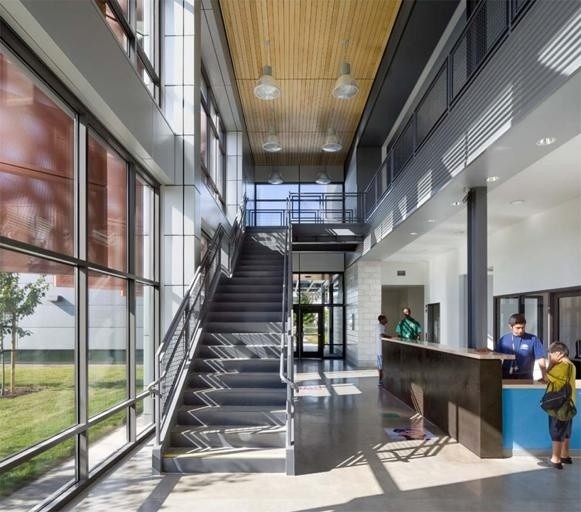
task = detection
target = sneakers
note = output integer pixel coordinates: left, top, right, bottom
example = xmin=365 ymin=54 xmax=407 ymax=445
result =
xmin=537 ymin=457 xmax=573 ymax=470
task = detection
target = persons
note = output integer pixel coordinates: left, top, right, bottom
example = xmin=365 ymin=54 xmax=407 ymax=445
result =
xmin=396 ymin=306 xmax=422 ymax=341
xmin=535 ymin=341 xmax=578 ymax=469
xmin=478 ymin=313 xmax=548 ymax=382
xmin=376 ymin=315 xmax=394 ymax=386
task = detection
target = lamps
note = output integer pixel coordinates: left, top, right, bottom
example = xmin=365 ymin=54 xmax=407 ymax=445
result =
xmin=332 ymin=40 xmax=360 ymax=100
xmin=321 ymin=127 xmax=343 ymax=153
xmin=267 ymin=163 xmax=283 ymax=185
xmin=262 ymin=126 xmax=283 ymax=152
xmin=253 ymin=41 xmax=281 ymax=100
xmin=314 ymin=159 xmax=331 ymax=185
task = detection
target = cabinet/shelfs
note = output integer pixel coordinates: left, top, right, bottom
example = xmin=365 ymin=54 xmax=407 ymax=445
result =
xmin=502 ymin=377 xmax=580 ymax=451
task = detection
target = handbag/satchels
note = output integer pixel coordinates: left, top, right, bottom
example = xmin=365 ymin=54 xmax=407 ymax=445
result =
xmin=539 ymin=383 xmax=577 ymax=421
